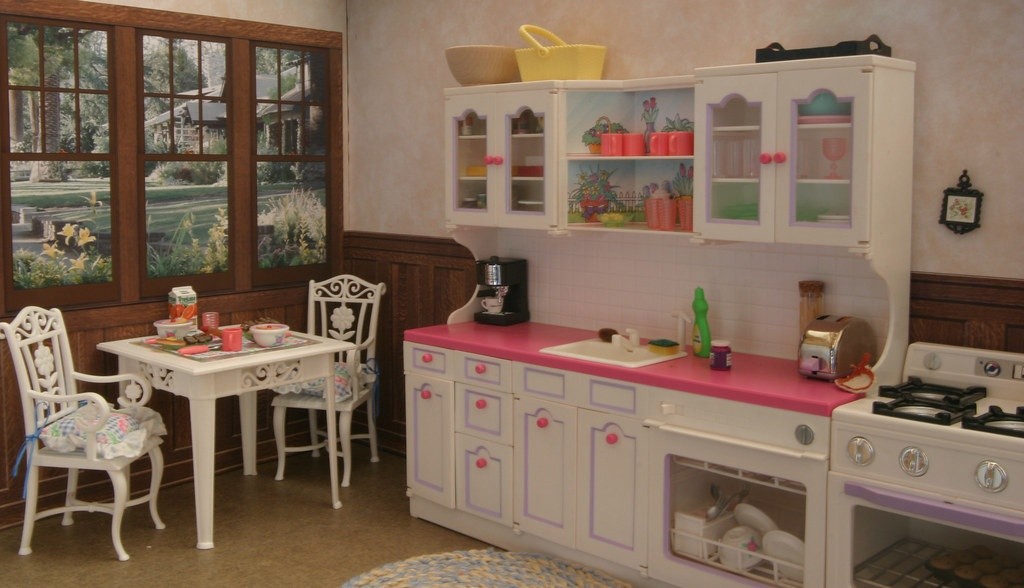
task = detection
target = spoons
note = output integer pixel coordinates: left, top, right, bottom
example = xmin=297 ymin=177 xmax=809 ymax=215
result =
xmin=704 ymin=507 xmax=719 ymax=520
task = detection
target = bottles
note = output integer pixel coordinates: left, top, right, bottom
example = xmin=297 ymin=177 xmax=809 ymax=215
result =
xmin=461 ymin=193 xmax=486 ymax=209
xmin=692 ymin=287 xmax=710 ymax=356
xmin=798 ymin=280 xmax=823 ymax=341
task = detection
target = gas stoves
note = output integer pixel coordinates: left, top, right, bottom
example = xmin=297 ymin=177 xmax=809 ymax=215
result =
xmin=832 ymin=343 xmax=1024 ymax=509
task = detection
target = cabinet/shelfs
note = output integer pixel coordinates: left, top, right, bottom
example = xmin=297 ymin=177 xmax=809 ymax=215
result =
xmin=403 ymin=52 xmax=917 ymax=588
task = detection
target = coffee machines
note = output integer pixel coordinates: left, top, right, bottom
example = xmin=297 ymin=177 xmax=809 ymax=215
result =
xmin=473 ymin=255 xmax=528 ymax=325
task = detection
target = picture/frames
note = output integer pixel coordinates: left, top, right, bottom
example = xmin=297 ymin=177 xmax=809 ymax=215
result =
xmin=941 ymin=168 xmax=985 ymax=236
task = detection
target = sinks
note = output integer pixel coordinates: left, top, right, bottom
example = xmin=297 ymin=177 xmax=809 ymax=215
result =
xmin=554 ymin=340 xmax=677 ymax=364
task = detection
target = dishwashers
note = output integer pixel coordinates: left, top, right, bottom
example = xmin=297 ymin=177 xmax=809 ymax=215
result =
xmin=641 ymin=376 xmax=831 ymax=588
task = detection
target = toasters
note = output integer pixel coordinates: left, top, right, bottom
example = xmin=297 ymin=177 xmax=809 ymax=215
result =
xmin=798 ymin=312 xmax=877 ymax=383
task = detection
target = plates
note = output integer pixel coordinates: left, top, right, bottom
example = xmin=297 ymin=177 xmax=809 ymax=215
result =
xmin=518 ymin=201 xmax=544 ymax=209
xmin=817 ymin=213 xmax=849 ymax=224
xmin=735 ymin=502 xmax=777 ymax=534
xmin=797 ymin=115 xmax=852 ymax=124
xmin=761 ymin=529 xmax=805 ymax=580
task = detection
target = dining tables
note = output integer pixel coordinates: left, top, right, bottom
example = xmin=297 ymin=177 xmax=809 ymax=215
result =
xmin=96 ymin=324 xmax=355 ymax=551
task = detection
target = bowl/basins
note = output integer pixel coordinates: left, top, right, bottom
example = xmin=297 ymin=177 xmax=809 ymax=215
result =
xmin=248 ymin=324 xmax=290 ymax=347
xmin=718 ymin=524 xmax=762 ymax=569
xmin=444 ymin=45 xmax=517 ymax=85
xmin=154 ymin=319 xmax=193 ymax=339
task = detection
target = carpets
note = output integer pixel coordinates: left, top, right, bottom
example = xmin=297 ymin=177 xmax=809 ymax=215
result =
xmin=340 ymin=547 xmax=637 ymax=588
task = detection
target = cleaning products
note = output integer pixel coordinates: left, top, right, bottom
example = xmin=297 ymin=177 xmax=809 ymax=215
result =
xmin=691 ymin=287 xmax=713 ymax=358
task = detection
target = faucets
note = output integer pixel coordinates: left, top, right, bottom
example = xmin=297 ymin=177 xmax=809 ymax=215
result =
xmin=611 ymin=328 xmax=640 ymax=352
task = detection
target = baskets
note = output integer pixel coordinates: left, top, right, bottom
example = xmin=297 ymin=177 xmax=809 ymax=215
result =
xmin=444 ymin=45 xmax=521 ymax=87
xmin=515 ymin=24 xmax=607 ymax=81
xmin=586 ymin=115 xmax=612 ymax=154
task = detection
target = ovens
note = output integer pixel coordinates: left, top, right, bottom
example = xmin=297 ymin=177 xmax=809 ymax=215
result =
xmin=826 ymin=471 xmax=1024 ymax=587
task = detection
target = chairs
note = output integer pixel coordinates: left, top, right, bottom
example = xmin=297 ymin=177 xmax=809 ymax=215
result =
xmin=271 ymin=274 xmax=387 ymax=488
xmin=0 ymin=306 xmax=170 ymax=562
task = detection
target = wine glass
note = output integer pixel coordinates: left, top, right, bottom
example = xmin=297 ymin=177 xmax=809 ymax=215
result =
xmin=822 ymin=137 xmax=847 ymax=179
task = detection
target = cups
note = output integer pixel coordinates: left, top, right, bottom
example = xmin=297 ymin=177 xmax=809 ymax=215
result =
xmin=646 ymin=132 xmax=669 ymax=155
xmin=645 ymin=198 xmax=693 ymax=232
xmin=600 ymin=133 xmax=622 ymax=155
xmin=202 ymin=312 xmax=219 ymax=328
xmin=712 ymin=135 xmax=815 ymax=179
xmin=222 ymin=328 xmax=242 ymax=351
xmin=621 ymin=134 xmax=646 ymax=156
xmin=669 ymin=132 xmax=694 ymax=156
